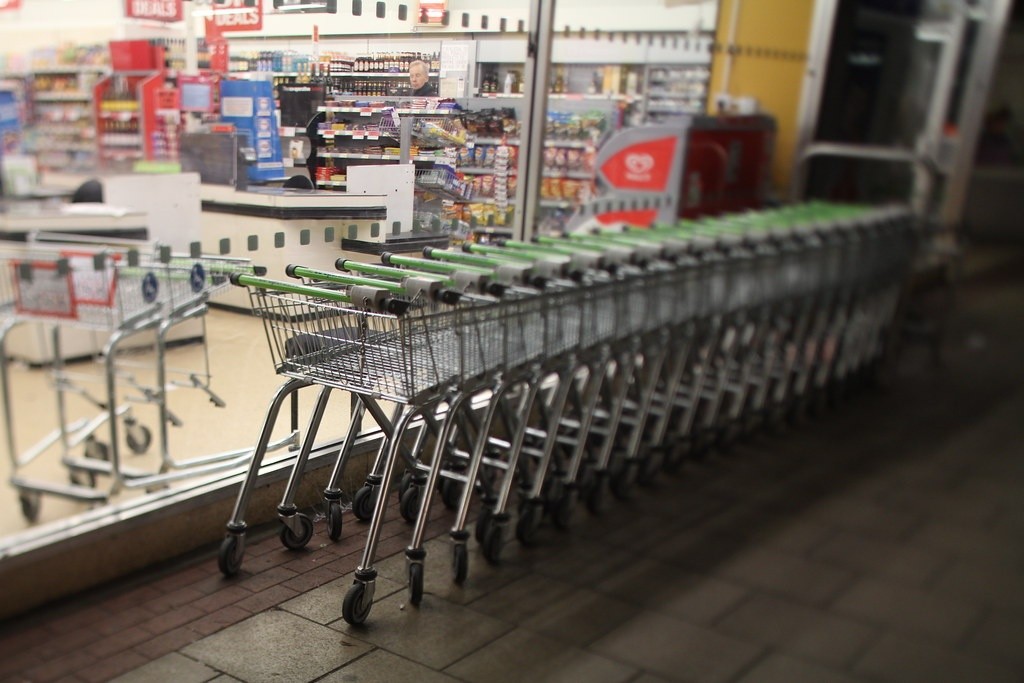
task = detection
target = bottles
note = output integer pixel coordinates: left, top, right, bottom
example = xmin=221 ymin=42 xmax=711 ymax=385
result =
xmin=198 ymin=39 xmax=210 ymax=68
xmin=555 ymin=65 xmax=564 ymax=93
xmin=164 ymin=36 xmax=186 ymax=67
xmin=504 ymin=70 xmax=517 ymax=93
xmin=483 ymin=72 xmax=498 ymax=93
xmin=228 ymin=50 xmax=440 ymax=100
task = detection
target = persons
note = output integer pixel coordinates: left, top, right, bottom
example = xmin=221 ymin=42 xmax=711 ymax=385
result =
xmin=409 ymin=60 xmax=439 ymax=96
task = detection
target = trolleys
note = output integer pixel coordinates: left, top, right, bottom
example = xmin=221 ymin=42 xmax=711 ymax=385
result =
xmin=0 ymin=229 xmax=258 ymax=527
xmin=220 ymin=203 xmax=928 ymax=630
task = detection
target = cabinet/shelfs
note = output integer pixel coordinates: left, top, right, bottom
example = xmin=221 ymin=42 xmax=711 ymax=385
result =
xmin=25 ymin=67 xmax=709 ymax=236
